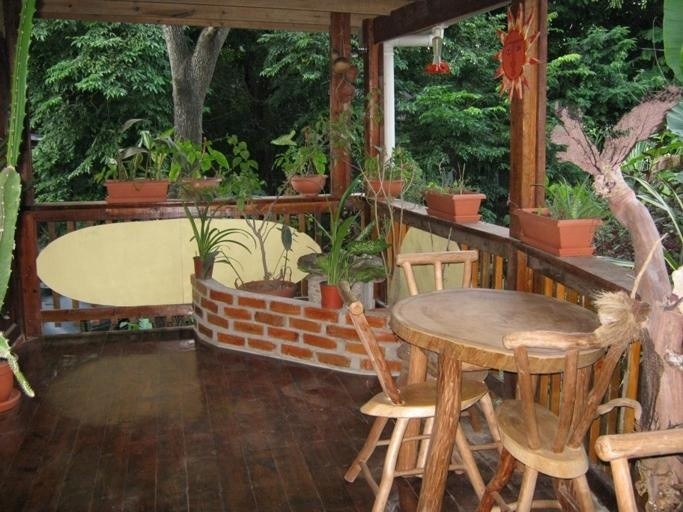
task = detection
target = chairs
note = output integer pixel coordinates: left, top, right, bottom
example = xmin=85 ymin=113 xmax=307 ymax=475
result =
xmin=343 ymin=303 xmax=488 ymax=512
xmin=594 ymin=429 xmax=683 ymax=512
xmin=396 ymin=250 xmax=504 ymax=456
xmin=479 ymin=340 xmax=629 ymax=512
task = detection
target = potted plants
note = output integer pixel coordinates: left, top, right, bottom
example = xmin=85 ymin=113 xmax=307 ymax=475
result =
xmin=104 ymin=118 xmax=178 ymax=206
xmin=362 ymin=145 xmax=411 ymax=198
xmin=272 ymin=112 xmax=332 ymax=196
xmin=424 ymin=155 xmax=487 ymax=226
xmin=0 ymin=0 xmax=37 ymax=412
xmin=182 ymin=199 xmax=256 ymax=282
xmin=513 ymin=176 xmax=605 ymax=258
xmin=303 ymin=170 xmax=384 ymax=313
xmin=232 ymin=190 xmax=299 ymax=300
xmin=178 ymin=131 xmax=225 ymax=206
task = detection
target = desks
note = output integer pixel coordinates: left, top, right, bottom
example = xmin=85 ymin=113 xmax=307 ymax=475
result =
xmin=388 ymin=289 xmax=611 ymax=512
